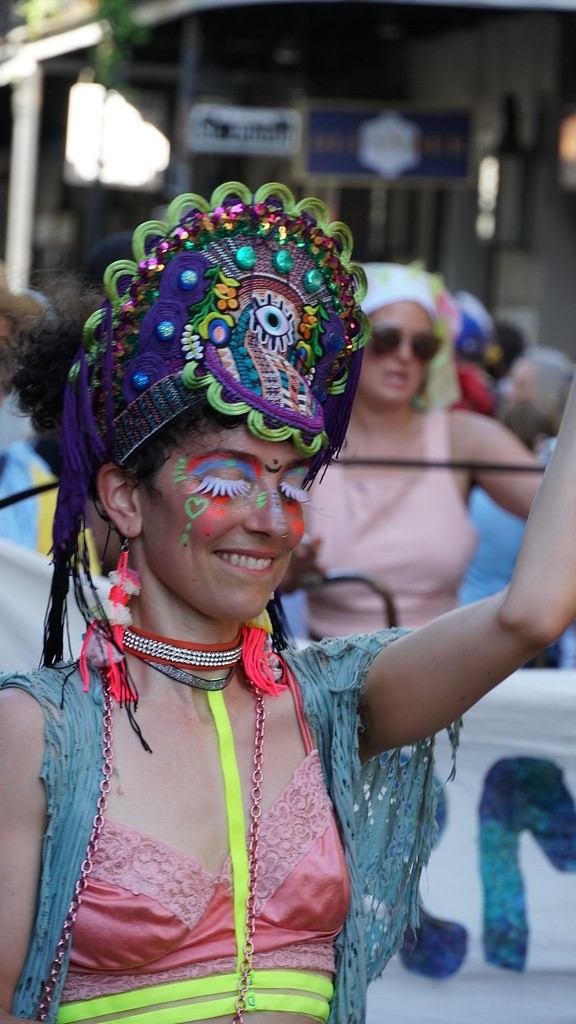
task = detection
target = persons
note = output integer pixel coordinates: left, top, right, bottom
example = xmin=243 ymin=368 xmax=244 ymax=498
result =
xmin=0 ymin=263 xmax=576 ymax=675
xmin=0 ymin=184 xmax=575 ymax=1024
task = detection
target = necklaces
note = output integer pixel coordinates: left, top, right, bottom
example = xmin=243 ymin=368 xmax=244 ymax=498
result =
xmin=117 ymin=625 xmax=243 ymax=689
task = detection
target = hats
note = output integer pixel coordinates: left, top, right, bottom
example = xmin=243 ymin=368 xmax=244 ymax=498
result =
xmin=358 ymin=262 xmax=462 ymax=343
xmin=452 ymin=290 xmax=496 ymax=357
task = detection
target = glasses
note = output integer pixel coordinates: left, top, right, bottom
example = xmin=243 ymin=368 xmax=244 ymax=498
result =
xmin=371 ymin=323 xmax=442 ymax=360
